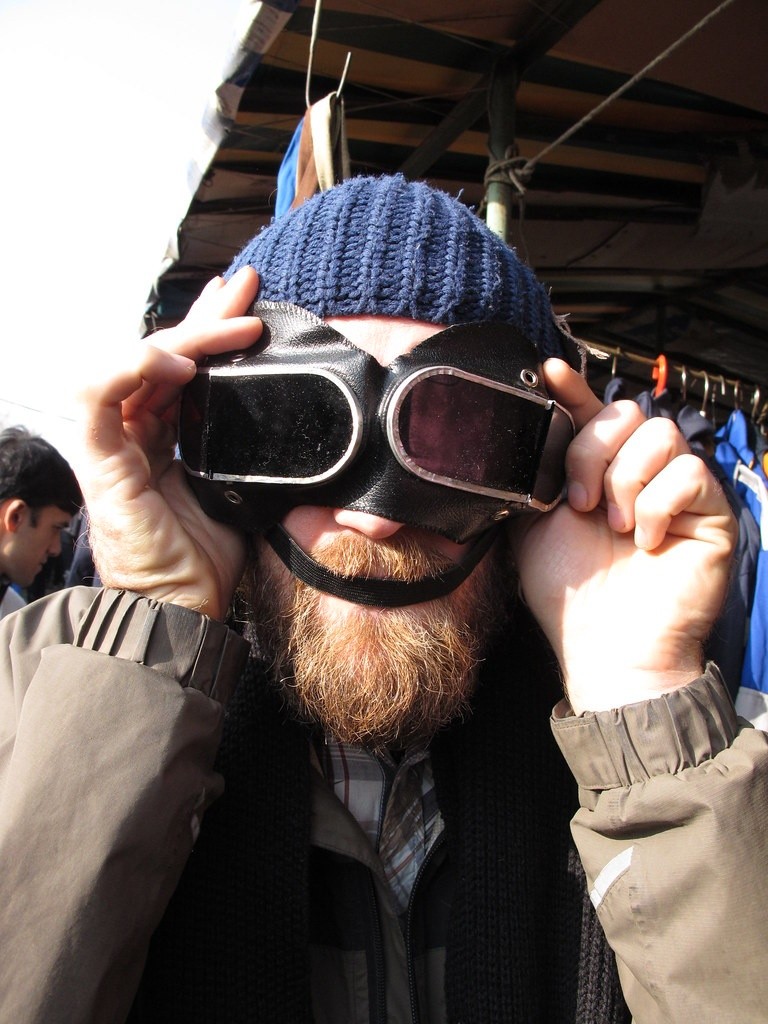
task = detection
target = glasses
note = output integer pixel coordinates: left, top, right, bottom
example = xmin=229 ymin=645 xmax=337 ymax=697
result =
xmin=177 ymin=301 xmax=575 ymax=542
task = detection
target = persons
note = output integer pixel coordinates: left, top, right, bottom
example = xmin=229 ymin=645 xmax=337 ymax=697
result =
xmin=0 ymin=172 xmax=768 ymax=1024
xmin=0 ymin=429 xmax=85 ymax=612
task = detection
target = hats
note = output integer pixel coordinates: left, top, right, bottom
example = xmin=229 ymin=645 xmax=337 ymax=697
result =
xmin=219 ymin=174 xmax=578 ymax=361
